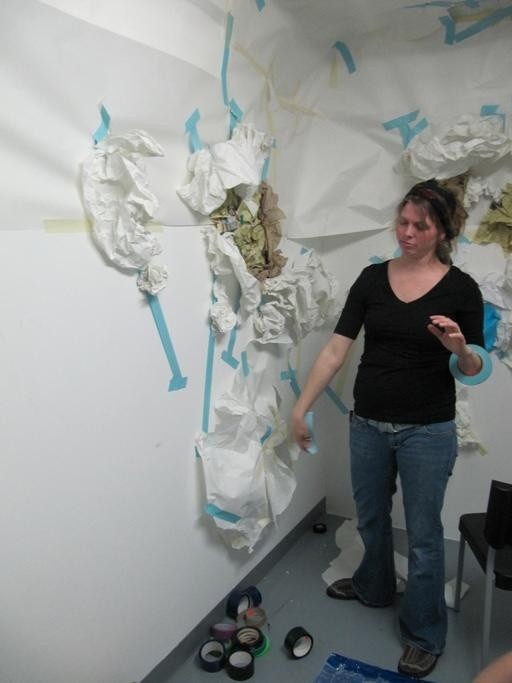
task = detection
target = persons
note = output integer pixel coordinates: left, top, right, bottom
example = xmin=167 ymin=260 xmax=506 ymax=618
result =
xmin=471 ymin=651 xmax=511 ymax=683
xmin=292 ymin=178 xmax=492 ymax=678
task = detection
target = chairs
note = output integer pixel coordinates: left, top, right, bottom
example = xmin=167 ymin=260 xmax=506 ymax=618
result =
xmin=439 ymin=474 xmax=512 ymax=682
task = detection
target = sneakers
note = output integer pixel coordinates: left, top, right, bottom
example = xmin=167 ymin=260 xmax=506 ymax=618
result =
xmin=326 ymin=578 xmax=358 ymax=601
xmin=398 ymin=646 xmax=438 ymax=676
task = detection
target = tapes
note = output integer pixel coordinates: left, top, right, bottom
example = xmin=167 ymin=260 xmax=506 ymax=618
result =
xmin=284 ymin=626 xmax=313 ymax=659
xmin=313 ymin=523 xmax=327 ymax=533
xmin=198 ymin=585 xmax=270 ymax=680
xmin=449 ymin=343 xmax=493 ymax=385
